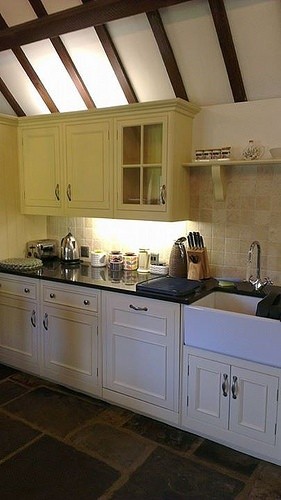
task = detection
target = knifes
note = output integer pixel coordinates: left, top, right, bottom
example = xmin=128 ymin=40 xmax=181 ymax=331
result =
xmin=188 ymin=231 xmax=205 ymax=251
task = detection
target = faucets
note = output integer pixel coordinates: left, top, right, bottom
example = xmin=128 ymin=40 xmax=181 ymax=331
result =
xmin=243 ymin=236 xmax=273 ymax=294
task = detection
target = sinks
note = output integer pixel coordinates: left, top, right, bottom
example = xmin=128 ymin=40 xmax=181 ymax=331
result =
xmin=182 ymin=285 xmax=281 ymax=369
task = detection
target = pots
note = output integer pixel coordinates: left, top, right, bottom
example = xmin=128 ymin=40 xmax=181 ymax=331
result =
xmin=26 ymin=239 xmax=58 ymax=260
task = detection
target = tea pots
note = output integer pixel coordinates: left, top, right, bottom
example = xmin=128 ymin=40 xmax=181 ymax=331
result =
xmin=61 ymin=233 xmax=80 ymax=264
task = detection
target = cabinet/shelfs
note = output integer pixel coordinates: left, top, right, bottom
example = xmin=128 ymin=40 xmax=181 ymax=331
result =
xmin=0 ymin=273 xmax=180 ymax=428
xmin=17 ymin=97 xmax=202 ymax=222
xmin=181 ymin=345 xmax=281 ymax=465
xmin=182 ymin=158 xmax=281 ymax=201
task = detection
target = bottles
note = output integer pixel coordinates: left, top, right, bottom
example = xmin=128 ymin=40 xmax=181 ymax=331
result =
xmin=242 ymin=139 xmax=260 ymax=161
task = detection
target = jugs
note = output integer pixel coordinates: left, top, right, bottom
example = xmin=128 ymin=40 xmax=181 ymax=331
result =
xmin=169 ymin=237 xmax=187 ymax=278
